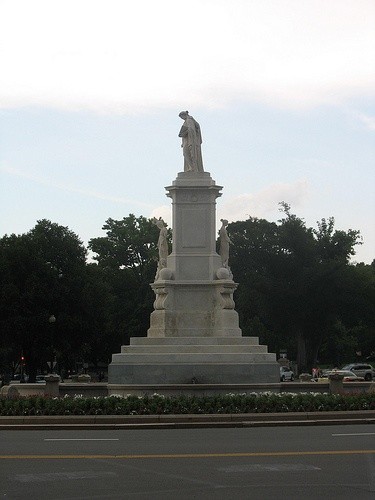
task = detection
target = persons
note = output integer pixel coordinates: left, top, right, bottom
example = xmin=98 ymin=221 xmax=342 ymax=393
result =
xmin=220 ymin=219 xmax=234 ymax=267
xmin=178 ymin=110 xmax=204 ymax=173
xmin=314 ymin=368 xmax=319 ymax=378
xmin=156 ymin=221 xmax=169 ymax=269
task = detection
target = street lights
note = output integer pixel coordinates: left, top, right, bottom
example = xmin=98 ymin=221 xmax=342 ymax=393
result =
xmin=20 ymin=357 xmax=26 ymax=383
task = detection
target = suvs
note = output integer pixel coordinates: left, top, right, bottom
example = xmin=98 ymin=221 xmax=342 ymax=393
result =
xmin=341 ymin=363 xmax=373 ymax=381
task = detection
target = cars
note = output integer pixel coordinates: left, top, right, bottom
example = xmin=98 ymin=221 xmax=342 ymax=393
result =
xmin=323 ymin=371 xmax=364 ymax=382
xmin=280 ymin=366 xmax=294 ymax=382
